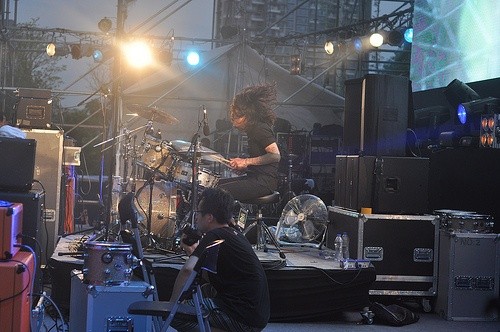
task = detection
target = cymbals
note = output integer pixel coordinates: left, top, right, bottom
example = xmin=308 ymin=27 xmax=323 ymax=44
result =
xmin=126 ymin=104 xmax=181 ymax=125
xmin=170 ymin=140 xmax=224 ymax=162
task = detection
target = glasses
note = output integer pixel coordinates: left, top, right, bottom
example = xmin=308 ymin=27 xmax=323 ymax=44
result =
xmin=192 ymin=209 xmax=209 ymax=216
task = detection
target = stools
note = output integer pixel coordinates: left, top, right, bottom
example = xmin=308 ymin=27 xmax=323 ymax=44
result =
xmin=237 ymin=189 xmax=285 ymax=259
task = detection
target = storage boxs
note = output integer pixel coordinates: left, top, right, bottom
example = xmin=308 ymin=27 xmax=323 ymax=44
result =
xmin=70 ymin=270 xmax=154 ymax=332
xmin=23 ymin=129 xmax=83 ymax=266
xmin=327 ymin=205 xmax=500 ymax=322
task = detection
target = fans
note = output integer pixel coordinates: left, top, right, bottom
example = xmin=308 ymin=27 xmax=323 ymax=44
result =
xmin=275 ymin=194 xmax=330 ymax=252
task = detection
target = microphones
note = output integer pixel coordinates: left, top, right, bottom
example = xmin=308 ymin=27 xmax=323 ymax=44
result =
xmin=203 ymin=105 xmax=209 ymax=136
xmin=166 ymin=140 xmax=187 ymax=159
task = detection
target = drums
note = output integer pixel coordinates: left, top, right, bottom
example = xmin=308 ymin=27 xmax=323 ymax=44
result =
xmin=110 ymin=179 xmax=178 ymax=241
xmin=136 ymin=135 xmax=181 ymax=180
xmin=83 ymin=241 xmax=133 ymax=286
xmin=167 ymin=159 xmax=219 ymax=192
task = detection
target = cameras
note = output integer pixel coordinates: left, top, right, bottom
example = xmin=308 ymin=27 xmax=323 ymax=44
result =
xmin=181 ymin=223 xmax=202 ymax=247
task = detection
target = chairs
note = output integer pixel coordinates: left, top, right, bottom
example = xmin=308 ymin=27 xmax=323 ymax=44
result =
xmin=127 ymin=238 xmax=225 ymax=332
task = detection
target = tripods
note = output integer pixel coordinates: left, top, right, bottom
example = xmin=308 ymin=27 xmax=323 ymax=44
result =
xmin=59 ymin=74 xmax=176 ymax=256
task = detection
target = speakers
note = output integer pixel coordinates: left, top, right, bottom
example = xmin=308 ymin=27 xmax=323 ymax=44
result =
xmin=0 ymin=136 xmax=46 ymax=332
xmin=13 ymin=98 xmax=51 ymax=128
xmin=334 ymin=74 xmax=430 ymax=215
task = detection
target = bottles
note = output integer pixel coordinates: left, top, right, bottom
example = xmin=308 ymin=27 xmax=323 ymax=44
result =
xmin=334 ymin=234 xmax=342 ymax=260
xmin=342 ymin=231 xmax=349 ymax=259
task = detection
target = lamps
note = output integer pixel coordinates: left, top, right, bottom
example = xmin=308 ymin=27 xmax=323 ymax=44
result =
xmin=46 ymin=34 xmax=114 ymax=64
xmin=289 ymin=54 xmax=305 ymax=75
xmin=273 ymin=119 xmax=292 ymax=132
xmin=216 ymin=119 xmax=232 ymax=130
xmin=221 ymin=26 xmax=238 ymax=39
xmin=99 ymin=17 xmax=112 ymax=32
xmin=324 ymin=25 xmax=413 ymax=54
xmin=409 ymin=79 xmax=500 ymax=153
xmin=154 ymin=52 xmax=173 ymax=65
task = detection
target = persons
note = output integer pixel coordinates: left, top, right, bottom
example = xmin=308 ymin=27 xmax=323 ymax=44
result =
xmin=215 ymin=81 xmax=281 ymax=232
xmin=162 ymin=187 xmax=269 ymax=332
